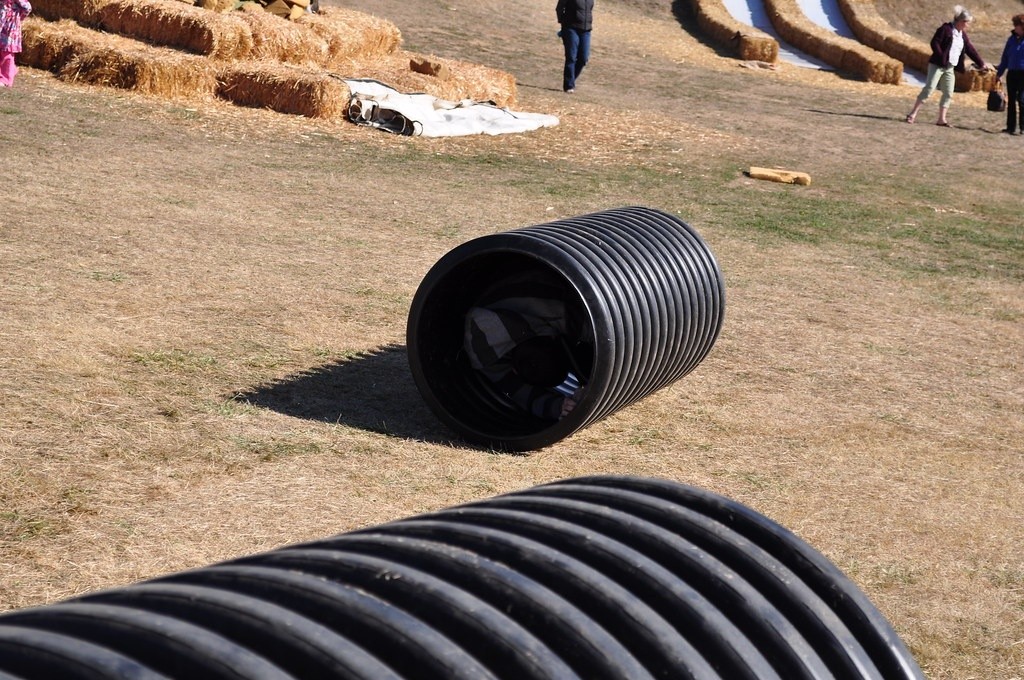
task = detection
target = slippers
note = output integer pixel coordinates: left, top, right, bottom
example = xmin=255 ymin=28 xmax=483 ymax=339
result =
xmin=936 ymin=122 xmax=952 ymax=127
xmin=907 ymin=115 xmax=914 ymax=123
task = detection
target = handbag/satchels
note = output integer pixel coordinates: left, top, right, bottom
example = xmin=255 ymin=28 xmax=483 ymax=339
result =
xmin=988 ymin=89 xmax=1005 ymax=111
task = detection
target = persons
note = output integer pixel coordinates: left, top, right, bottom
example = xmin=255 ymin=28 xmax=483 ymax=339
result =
xmin=555 ymin=0 xmax=594 ymax=94
xmin=987 ymin=14 xmax=1024 ymax=135
xmin=0 ymin=0 xmax=31 ymax=86
xmin=906 ymin=4 xmax=992 ymax=128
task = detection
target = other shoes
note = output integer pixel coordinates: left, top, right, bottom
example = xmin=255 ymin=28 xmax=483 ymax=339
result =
xmin=567 ymin=89 xmax=574 ymax=93
xmin=1002 ymin=129 xmax=1014 ymax=135
xmin=1020 ymin=129 xmax=1024 ymax=134
